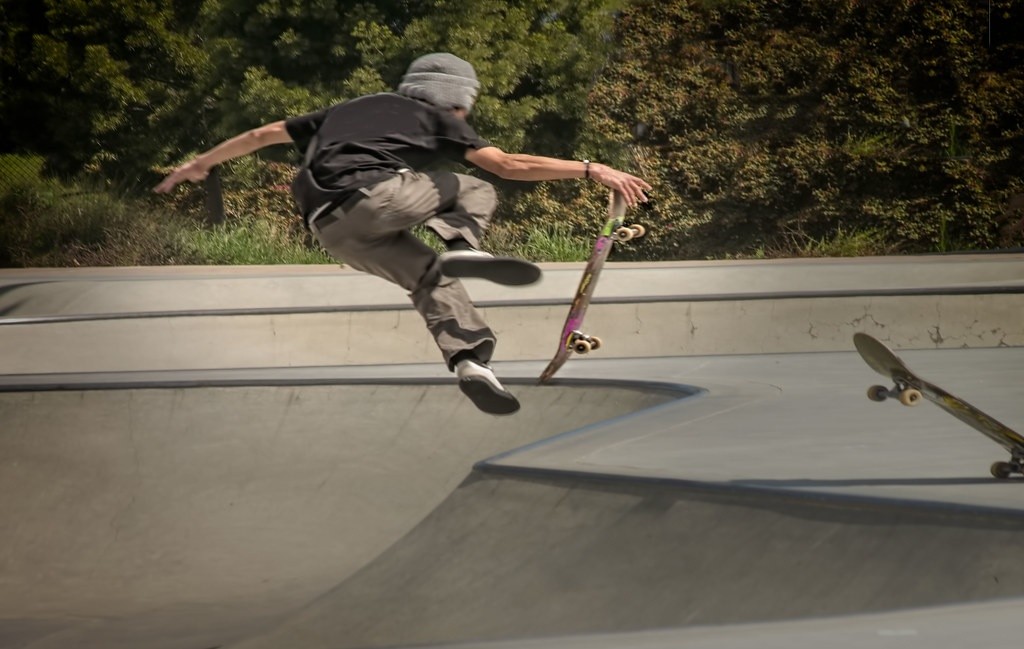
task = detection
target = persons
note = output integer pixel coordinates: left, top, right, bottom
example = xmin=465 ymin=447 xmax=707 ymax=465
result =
xmin=154 ymin=54 xmax=653 ymax=416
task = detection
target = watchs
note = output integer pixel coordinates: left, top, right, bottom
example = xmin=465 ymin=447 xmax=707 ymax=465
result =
xmin=583 ymin=159 xmax=590 ymax=179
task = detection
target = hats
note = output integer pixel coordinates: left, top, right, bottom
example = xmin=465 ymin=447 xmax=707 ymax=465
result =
xmin=400 ymin=53 xmax=480 ymax=115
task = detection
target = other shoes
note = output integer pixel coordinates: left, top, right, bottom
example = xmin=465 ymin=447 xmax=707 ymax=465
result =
xmin=458 ymin=359 xmax=520 ymax=415
xmin=439 ymin=249 xmax=542 ymax=286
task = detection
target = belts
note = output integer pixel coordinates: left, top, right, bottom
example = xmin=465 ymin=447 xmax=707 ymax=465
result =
xmin=306 ymin=185 xmax=373 ymax=236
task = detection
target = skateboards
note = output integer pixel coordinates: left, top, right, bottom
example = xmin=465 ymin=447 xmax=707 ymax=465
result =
xmin=850 ymin=326 xmax=1023 ymax=477
xmin=534 ymin=187 xmax=646 ymax=389
